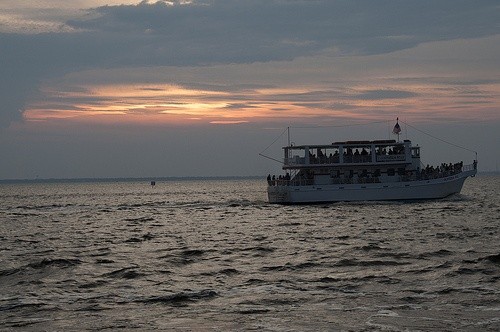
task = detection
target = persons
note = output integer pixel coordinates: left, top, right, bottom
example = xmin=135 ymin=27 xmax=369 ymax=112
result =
xmin=427 ymin=161 xmax=463 ymax=176
xmin=343 ymin=148 xmax=372 ymax=161
xmin=308 ymin=172 xmax=313 ymax=185
xmin=267 ymin=173 xmax=290 ymax=186
xmin=382 ymin=146 xmax=404 ymax=159
xmin=309 ymin=151 xmax=339 ymax=163
xmin=373 ymin=168 xmax=402 ymax=183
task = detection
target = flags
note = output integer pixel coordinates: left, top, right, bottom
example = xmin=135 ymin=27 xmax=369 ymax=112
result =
xmin=393 ymin=123 xmax=401 ymax=134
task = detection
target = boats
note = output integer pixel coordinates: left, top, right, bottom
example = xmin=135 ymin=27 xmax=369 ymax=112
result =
xmin=259 ymin=116 xmax=479 ymax=206
